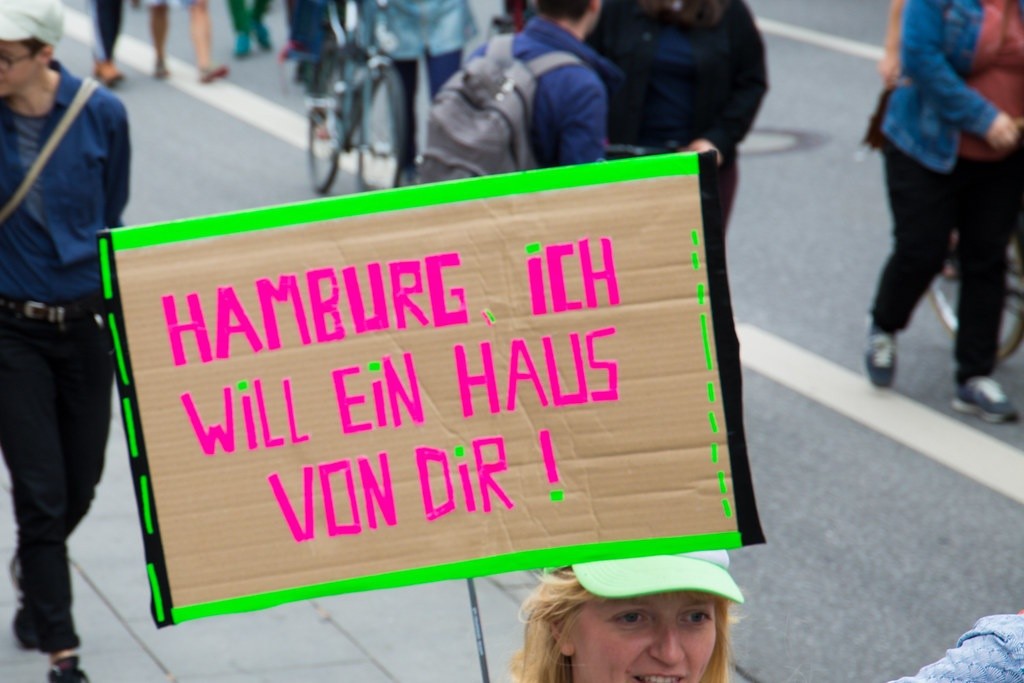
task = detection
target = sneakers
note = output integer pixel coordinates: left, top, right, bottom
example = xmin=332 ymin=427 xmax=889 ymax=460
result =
xmin=952 ymin=375 xmax=1017 ymax=423
xmin=866 ymin=321 xmax=897 ymax=386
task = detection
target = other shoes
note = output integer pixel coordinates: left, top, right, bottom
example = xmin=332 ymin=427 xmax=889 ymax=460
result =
xmin=47 ymin=655 xmax=90 ymax=683
xmin=234 ymin=33 xmax=253 ymax=57
xmin=8 ymin=555 xmax=40 ymax=650
xmin=253 ymin=21 xmax=271 ymax=48
xmin=156 ymin=60 xmax=168 ymax=76
xmin=202 ymin=66 xmax=226 ymax=81
xmin=94 ymin=61 xmax=124 ymax=84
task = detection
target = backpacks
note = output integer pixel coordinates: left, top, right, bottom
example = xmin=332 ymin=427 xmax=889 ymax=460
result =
xmin=423 ymin=29 xmax=583 ymax=185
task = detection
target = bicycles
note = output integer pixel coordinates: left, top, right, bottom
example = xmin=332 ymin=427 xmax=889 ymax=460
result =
xmin=303 ymin=36 xmax=407 ymax=197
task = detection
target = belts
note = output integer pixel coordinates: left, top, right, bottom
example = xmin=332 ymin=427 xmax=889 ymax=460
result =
xmin=1 ymin=301 xmax=80 ymax=329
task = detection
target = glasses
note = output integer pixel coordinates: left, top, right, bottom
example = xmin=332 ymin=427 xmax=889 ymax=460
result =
xmin=0 ymin=51 xmax=34 ymax=73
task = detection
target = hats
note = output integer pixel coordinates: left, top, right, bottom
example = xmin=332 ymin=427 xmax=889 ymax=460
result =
xmin=0 ymin=0 xmax=63 ymax=48
xmin=542 ymin=550 xmax=744 ymax=605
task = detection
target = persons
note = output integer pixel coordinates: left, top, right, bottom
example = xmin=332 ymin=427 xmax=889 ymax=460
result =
xmin=886 ymin=608 xmax=1024 ymax=683
xmin=131 ymin=0 xmax=229 ymax=84
xmin=0 ymin=0 xmax=131 ymax=683
xmin=92 ymin=0 xmax=125 ymax=87
xmin=226 ymin=0 xmax=273 ymax=58
xmin=508 ymin=550 xmax=744 ymax=683
xmin=863 ymin=0 xmax=1024 ymax=423
xmin=580 ymin=1 xmax=768 ymax=232
xmin=280 ymin=0 xmax=478 ymax=186
xmin=469 ymin=0 xmax=628 ymax=170
